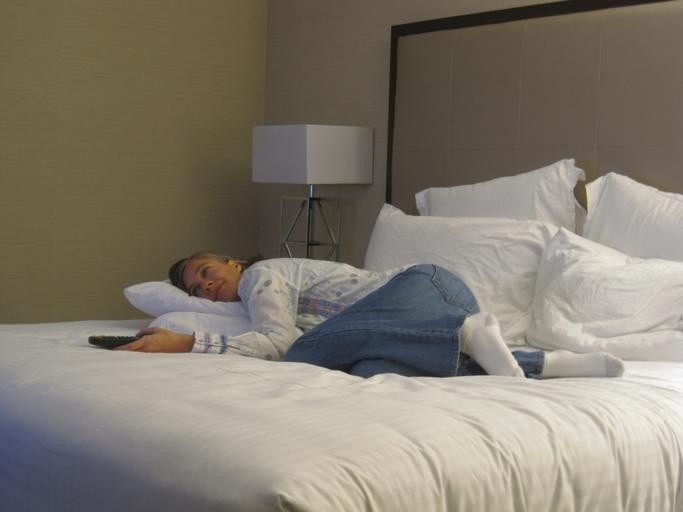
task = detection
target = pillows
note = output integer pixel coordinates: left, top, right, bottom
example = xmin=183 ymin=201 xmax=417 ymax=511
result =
xmin=362 ymin=203 xmax=557 ymax=349
xmin=527 ymin=225 xmax=683 ymax=363
xmin=413 ymin=159 xmax=588 ymax=232
xmin=582 ymin=170 xmax=683 ymax=264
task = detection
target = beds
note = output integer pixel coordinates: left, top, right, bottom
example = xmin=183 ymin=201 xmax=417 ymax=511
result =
xmin=0 ymin=318 xmax=682 ymax=511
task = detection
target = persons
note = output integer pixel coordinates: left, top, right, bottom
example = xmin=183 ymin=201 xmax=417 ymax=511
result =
xmin=113 ymin=251 xmax=627 ymax=378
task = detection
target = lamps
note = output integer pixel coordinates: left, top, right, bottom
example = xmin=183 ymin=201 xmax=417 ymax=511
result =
xmin=252 ymin=124 xmax=374 ymax=260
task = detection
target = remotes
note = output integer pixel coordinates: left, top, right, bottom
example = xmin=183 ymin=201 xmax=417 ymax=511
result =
xmin=89 ymin=334 xmax=141 ymax=347
